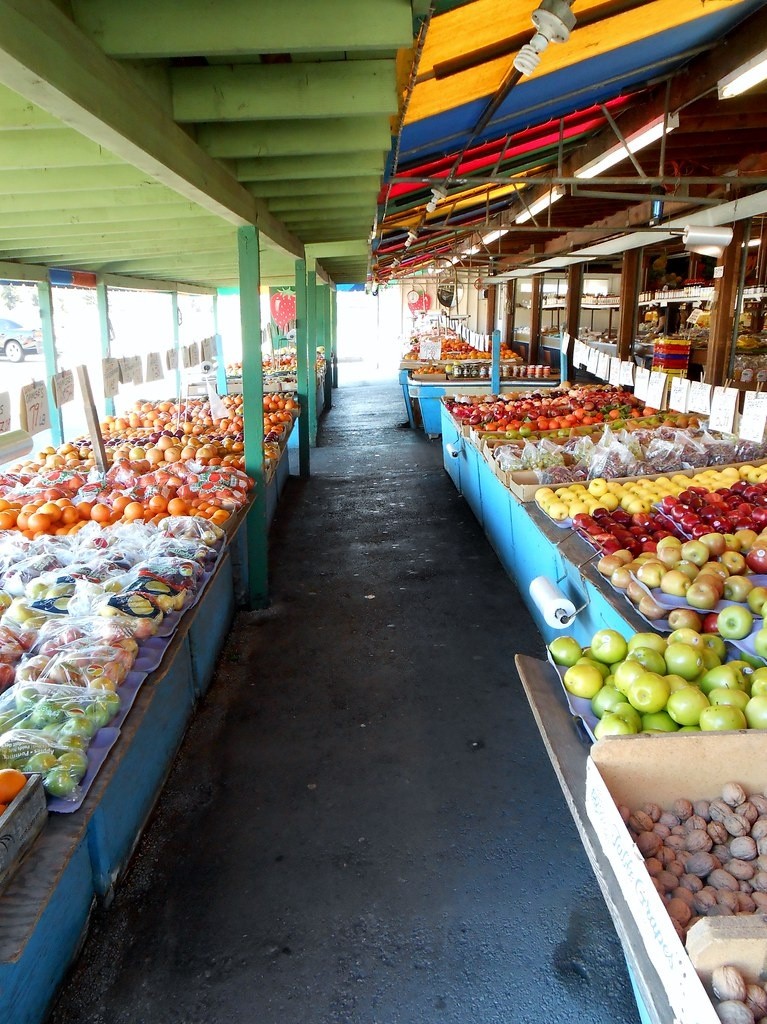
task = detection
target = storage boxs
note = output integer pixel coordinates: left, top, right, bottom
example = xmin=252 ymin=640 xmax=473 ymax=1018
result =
xmin=188 ymin=375 xmax=299 ymax=394
xmin=459 ymin=406 xmax=767 ymax=503
xmin=584 ymin=728 xmax=766 ymax=1024
xmin=0 ymin=771 xmax=49 ymax=901
xmin=412 ymin=372 xmax=446 ymax=380
xmin=651 ymin=337 xmax=692 ymax=392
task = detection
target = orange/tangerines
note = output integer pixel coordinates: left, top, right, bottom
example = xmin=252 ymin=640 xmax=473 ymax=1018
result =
xmin=442 ymin=349 xmax=518 ymax=359
xmin=0 ymin=497 xmax=229 ymax=541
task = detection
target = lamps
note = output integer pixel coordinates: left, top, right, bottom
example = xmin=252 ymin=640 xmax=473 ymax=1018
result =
xmin=512 ymin=0 xmax=577 ymax=76
xmin=717 ymin=48 xmax=767 ymax=100
xmin=574 ymin=111 xmax=679 ymax=183
xmin=405 ymin=229 xmax=418 ymax=247
xmin=439 ymin=245 xmax=481 ymax=274
xmin=391 ymin=258 xmax=401 ymax=268
xmin=514 ymin=185 xmax=566 ymax=224
xmin=482 ymin=223 xmax=511 ymax=245
xmin=426 ymin=184 xmax=448 ymax=213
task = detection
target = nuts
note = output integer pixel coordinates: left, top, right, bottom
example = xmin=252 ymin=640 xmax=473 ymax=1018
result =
xmin=709 ymin=965 xmax=767 ymax=1024
xmin=618 ymin=783 xmax=767 ymax=944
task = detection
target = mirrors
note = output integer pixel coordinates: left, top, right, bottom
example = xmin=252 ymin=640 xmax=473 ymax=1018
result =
xmin=437 ymin=278 xmax=464 ymax=307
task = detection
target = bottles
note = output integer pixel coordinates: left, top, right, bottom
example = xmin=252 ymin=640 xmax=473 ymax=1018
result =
xmin=638 ymin=278 xmax=715 ymax=302
xmin=736 ymin=284 xmax=767 ymax=295
xmin=521 ymin=295 xmax=532 ymax=306
xmin=439 ymin=361 xmax=551 ymax=379
xmin=543 ymin=293 xmax=566 ymax=305
xmin=581 ymin=292 xmax=620 ymax=304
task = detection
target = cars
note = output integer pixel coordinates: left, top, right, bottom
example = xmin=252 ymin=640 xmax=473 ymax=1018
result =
xmin=0 ymin=316 xmax=45 ymax=363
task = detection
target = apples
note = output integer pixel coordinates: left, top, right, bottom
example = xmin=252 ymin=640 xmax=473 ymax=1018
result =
xmin=447 ymin=385 xmax=767 ymax=738
xmin=0 ymin=580 xmax=154 ymax=797
xmin=0 ymin=395 xmax=295 ymax=497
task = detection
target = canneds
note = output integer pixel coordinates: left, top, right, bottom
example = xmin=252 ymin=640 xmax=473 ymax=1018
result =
xmin=454 ymin=365 xmax=550 ymax=379
